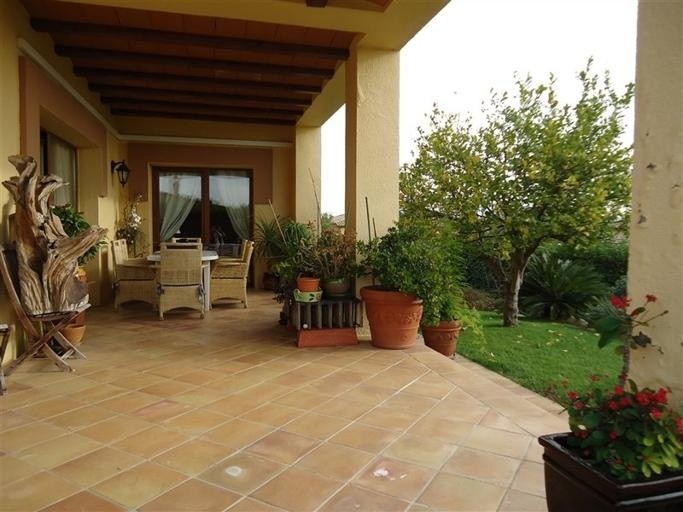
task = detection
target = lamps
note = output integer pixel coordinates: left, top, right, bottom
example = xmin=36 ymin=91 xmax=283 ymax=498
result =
xmin=109 ymin=159 xmax=130 ymax=189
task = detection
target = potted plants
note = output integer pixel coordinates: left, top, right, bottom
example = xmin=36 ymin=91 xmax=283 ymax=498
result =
xmin=291 ymin=229 xmax=351 ymax=300
xmin=253 ymin=209 xmax=289 ymax=290
xmin=351 ymin=212 xmax=487 ymax=361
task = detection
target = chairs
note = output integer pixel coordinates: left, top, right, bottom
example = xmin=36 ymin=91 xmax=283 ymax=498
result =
xmin=0 ymin=243 xmax=91 ymax=375
xmin=109 ymin=237 xmax=255 ymax=322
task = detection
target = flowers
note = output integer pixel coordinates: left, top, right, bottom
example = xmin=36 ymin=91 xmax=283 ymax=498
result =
xmin=557 ymin=287 xmax=683 ymax=486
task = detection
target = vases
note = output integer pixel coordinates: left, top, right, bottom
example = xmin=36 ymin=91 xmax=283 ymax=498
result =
xmin=535 ymin=430 xmax=682 ymax=512
